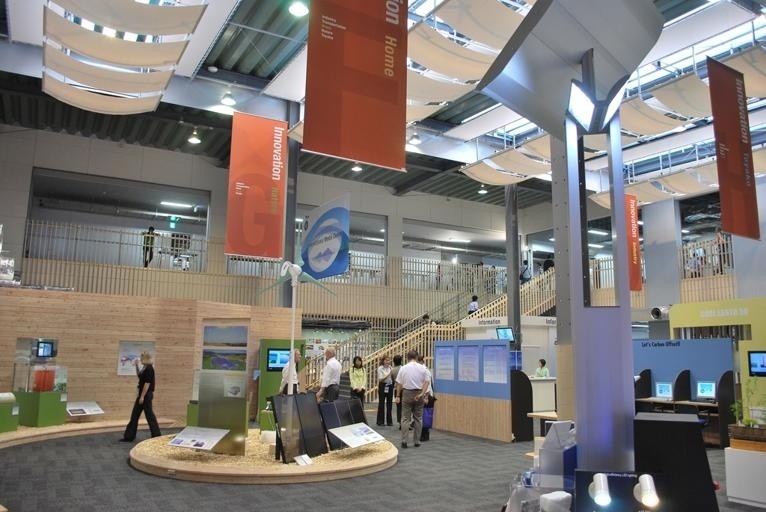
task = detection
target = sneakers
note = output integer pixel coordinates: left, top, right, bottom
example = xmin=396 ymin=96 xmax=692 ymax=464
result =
xmin=378 ymin=420 xmax=416 ymax=431
xmin=400 ymin=435 xmax=430 ymax=448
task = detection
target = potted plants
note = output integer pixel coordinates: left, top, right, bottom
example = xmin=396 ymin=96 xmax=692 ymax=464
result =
xmin=726 ymin=374 xmax=766 ymax=443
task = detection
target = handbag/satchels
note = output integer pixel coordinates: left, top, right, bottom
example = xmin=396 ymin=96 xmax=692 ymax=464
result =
xmin=520 ymin=275 xmax=523 ymax=280
xmin=421 ymin=407 xmax=434 ymax=428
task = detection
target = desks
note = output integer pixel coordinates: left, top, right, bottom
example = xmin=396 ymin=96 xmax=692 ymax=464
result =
xmin=638 ymin=395 xmax=674 ymax=412
xmin=524 ymin=412 xmax=559 ymax=464
xmin=675 ymin=399 xmax=718 ymax=418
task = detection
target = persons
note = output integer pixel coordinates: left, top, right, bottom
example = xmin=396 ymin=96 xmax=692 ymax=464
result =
xmin=140 ymin=226 xmax=161 ymax=268
xmin=349 ymin=356 xmax=368 ymax=410
xmin=685 ymin=252 xmax=704 ymax=278
xmin=533 ymin=262 xmax=544 ymax=276
xmin=467 ymin=294 xmax=482 ymax=318
xmin=535 ymin=358 xmax=550 ymax=376
xmin=395 ymin=350 xmax=432 ymax=449
xmin=278 ymin=348 xmax=301 ymax=395
xmin=315 ymin=347 xmax=342 ymax=402
xmin=390 ymin=355 xmax=413 ymax=431
xmin=117 ymin=350 xmax=161 ymax=442
xmin=543 ymin=254 xmax=554 ymax=271
xmin=520 ymin=260 xmax=533 ymax=285
xmin=422 ymin=314 xmax=438 ymax=341
xmin=716 ymin=226 xmax=730 ymax=275
xmin=376 ymin=354 xmax=395 ymax=427
xmin=416 ymin=355 xmax=438 ymax=441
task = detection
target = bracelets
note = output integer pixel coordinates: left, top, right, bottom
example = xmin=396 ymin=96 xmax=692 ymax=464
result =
xmin=396 ymin=395 xmax=401 ymax=397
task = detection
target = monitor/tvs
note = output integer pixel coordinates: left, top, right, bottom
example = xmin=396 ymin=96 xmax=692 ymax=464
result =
xmin=496 ymin=327 xmax=515 ymax=342
xmin=696 ymin=382 xmax=717 ymax=399
xmin=37 ymin=341 xmax=53 ymax=357
xmin=746 ymin=350 xmax=766 ymax=376
xmin=655 ymin=383 xmax=673 ymax=399
xmin=267 ymin=348 xmax=297 ymax=372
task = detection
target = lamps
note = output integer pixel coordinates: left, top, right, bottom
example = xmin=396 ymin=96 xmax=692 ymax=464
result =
xmin=220 ymin=83 xmax=238 ymax=107
xmin=407 ymin=129 xmax=422 ymax=145
xmin=186 ymin=126 xmax=202 ymax=145
xmin=566 ymin=48 xmax=633 ymax=133
xmin=584 ymin=470 xmax=662 ymax=507
